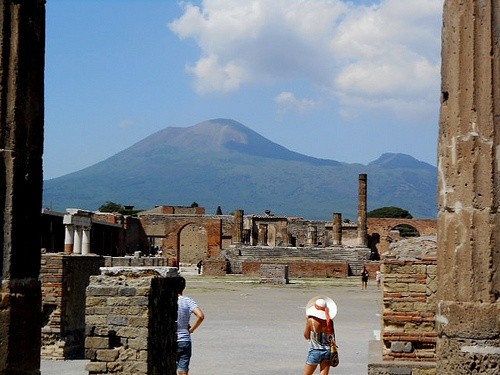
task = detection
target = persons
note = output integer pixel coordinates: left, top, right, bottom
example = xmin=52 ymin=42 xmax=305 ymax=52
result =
xmin=197 ymin=259 xmax=205 ymax=274
xmin=177 ymin=276 xmax=205 ymax=375
xmin=304 ymin=295 xmax=337 ymax=375
xmin=374 ymin=266 xmax=380 ymax=286
xmin=360 ymin=266 xmax=369 ymax=290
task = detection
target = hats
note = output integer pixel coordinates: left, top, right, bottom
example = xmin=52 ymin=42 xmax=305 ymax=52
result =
xmin=306 ymin=295 xmax=338 ymax=320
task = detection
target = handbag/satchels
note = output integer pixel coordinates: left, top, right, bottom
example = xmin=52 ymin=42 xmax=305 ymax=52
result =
xmin=330 ymin=340 xmax=339 ymax=368
